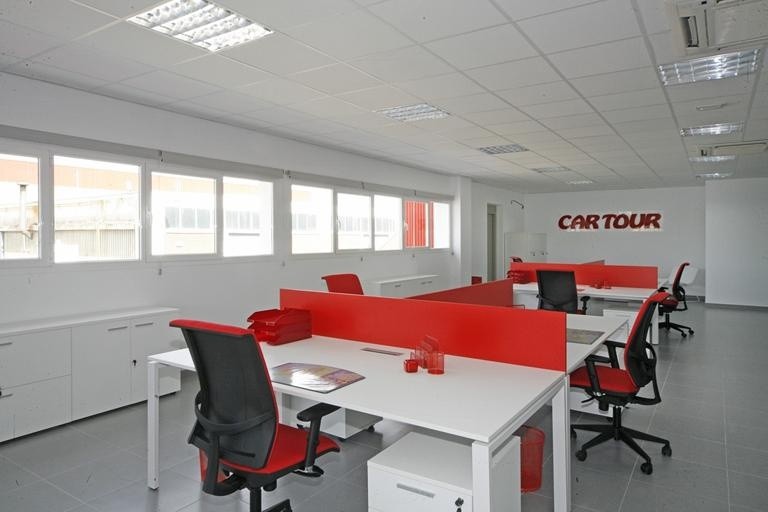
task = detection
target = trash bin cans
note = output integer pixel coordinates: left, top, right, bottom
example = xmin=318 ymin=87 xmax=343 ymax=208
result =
xmin=199 ymin=448 xmax=230 ymax=483
xmin=512 ymin=424 xmax=545 ymax=494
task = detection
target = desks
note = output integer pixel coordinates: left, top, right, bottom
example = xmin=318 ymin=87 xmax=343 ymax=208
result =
xmin=506 ymin=281 xmax=659 ymax=345
xmin=144 ymin=335 xmax=575 ymax=511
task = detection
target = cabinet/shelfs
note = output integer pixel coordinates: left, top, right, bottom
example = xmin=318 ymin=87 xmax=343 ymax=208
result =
xmin=377 ymin=274 xmax=441 ymax=298
xmin=1 ymin=307 xmax=180 ymax=441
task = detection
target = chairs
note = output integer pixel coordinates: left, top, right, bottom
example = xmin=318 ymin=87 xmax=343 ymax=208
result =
xmin=168 ymin=320 xmax=340 ymax=512
xmin=321 ymin=272 xmax=364 ymax=295
xmin=535 ymin=261 xmax=692 ymax=477
